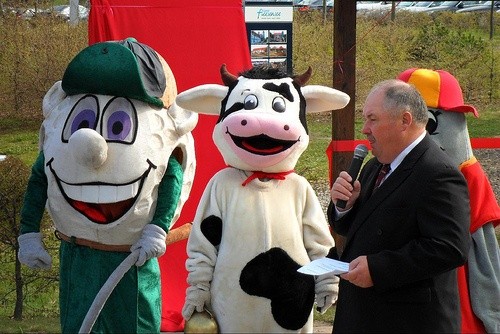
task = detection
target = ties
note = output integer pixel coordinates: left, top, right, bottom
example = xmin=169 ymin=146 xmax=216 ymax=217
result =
xmin=372 ymin=164 xmax=390 ymax=195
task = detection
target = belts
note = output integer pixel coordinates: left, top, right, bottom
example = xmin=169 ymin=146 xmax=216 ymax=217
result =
xmin=58 ymin=232 xmax=134 ymax=252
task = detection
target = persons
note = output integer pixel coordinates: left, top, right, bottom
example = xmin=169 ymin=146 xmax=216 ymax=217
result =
xmin=327 ymin=79 xmax=471 ymax=334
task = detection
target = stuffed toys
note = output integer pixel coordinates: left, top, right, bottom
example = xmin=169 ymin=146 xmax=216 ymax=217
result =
xmin=174 ymin=62 xmax=351 ymax=334
xmin=17 ymin=37 xmax=199 ymax=334
xmin=395 ymin=67 xmax=500 ymax=334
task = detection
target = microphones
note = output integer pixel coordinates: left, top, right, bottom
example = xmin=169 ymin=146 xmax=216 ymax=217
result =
xmin=335 ymin=143 xmax=368 ymax=209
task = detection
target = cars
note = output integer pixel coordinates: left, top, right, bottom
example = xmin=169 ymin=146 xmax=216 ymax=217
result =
xmin=290 ymin=0 xmax=500 ymax=15
xmin=47 ymin=4 xmax=89 ymax=20
xmin=4 ymin=7 xmax=43 ymax=16
